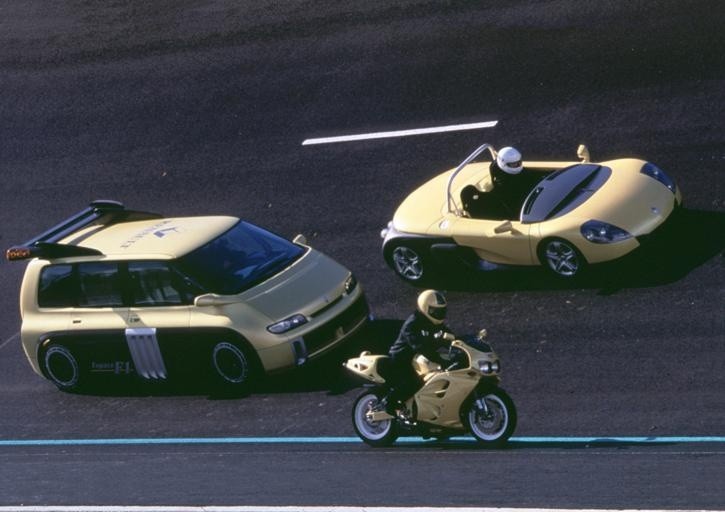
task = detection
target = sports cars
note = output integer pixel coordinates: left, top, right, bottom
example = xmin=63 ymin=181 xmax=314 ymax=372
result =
xmin=6 ymin=195 xmax=369 ymax=397
xmin=381 ymin=142 xmax=685 ymax=288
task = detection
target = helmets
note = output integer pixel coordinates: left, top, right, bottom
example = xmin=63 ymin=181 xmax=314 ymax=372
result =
xmin=417 ymin=289 xmax=448 ymax=325
xmin=496 ymin=147 xmax=523 ymax=175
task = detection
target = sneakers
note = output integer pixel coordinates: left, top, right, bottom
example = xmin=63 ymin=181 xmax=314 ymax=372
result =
xmin=382 ymin=398 xmax=397 ymax=416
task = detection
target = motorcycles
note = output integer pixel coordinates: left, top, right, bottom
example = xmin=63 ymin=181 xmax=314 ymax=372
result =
xmin=342 ymin=328 xmax=517 ymax=452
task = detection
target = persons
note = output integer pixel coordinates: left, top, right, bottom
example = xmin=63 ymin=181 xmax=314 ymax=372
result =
xmin=495 ymin=145 xmax=539 ymax=215
xmin=205 ymin=242 xmax=251 ymax=269
xmin=383 ymin=288 xmax=465 ymax=419
